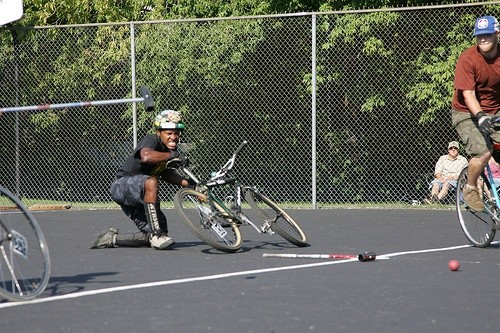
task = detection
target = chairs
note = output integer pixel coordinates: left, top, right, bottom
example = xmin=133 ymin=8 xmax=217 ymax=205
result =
xmin=425 ymin=171 xmax=456 ymax=203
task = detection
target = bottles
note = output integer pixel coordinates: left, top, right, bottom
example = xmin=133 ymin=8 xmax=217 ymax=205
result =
xmin=228 ymin=196 xmax=238 ymax=210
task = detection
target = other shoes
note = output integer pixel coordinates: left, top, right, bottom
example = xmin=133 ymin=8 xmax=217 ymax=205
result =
xmin=424 ymin=193 xmax=440 ymax=205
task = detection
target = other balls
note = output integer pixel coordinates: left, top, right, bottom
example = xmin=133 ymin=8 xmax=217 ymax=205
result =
xmin=450 ymin=259 xmax=460 ymax=271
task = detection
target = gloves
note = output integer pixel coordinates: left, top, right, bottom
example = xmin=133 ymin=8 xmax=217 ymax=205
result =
xmin=170 ymin=151 xmax=189 ymax=166
xmin=475 ymin=111 xmax=493 ymax=134
xmin=188 ymin=175 xmax=200 ymax=188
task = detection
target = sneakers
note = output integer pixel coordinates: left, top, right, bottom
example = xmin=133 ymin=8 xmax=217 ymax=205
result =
xmin=149 ymin=233 xmax=175 ymax=249
xmin=87 ymin=225 xmax=119 ymax=249
xmin=461 ymin=184 xmax=485 ymax=211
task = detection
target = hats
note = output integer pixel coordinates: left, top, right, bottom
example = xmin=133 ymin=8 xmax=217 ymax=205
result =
xmin=472 ymin=16 xmax=499 ymax=38
xmin=447 ymin=141 xmax=460 ymax=149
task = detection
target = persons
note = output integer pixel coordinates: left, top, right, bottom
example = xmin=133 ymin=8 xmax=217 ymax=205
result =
xmin=450 ymin=16 xmax=500 ymax=211
xmin=422 ymin=141 xmax=469 ymax=204
xmin=88 ymin=110 xmax=201 ymax=248
xmin=477 ymin=157 xmax=500 ymax=202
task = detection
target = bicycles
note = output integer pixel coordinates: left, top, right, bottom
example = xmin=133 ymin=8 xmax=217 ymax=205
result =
xmin=456 ymin=116 xmax=500 ymax=248
xmin=166 ymin=140 xmax=307 ymax=252
xmin=0 ymin=184 xmax=52 ymax=302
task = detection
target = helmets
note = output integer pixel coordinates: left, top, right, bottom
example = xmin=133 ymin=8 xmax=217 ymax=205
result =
xmin=153 ymin=110 xmax=185 ymax=130
